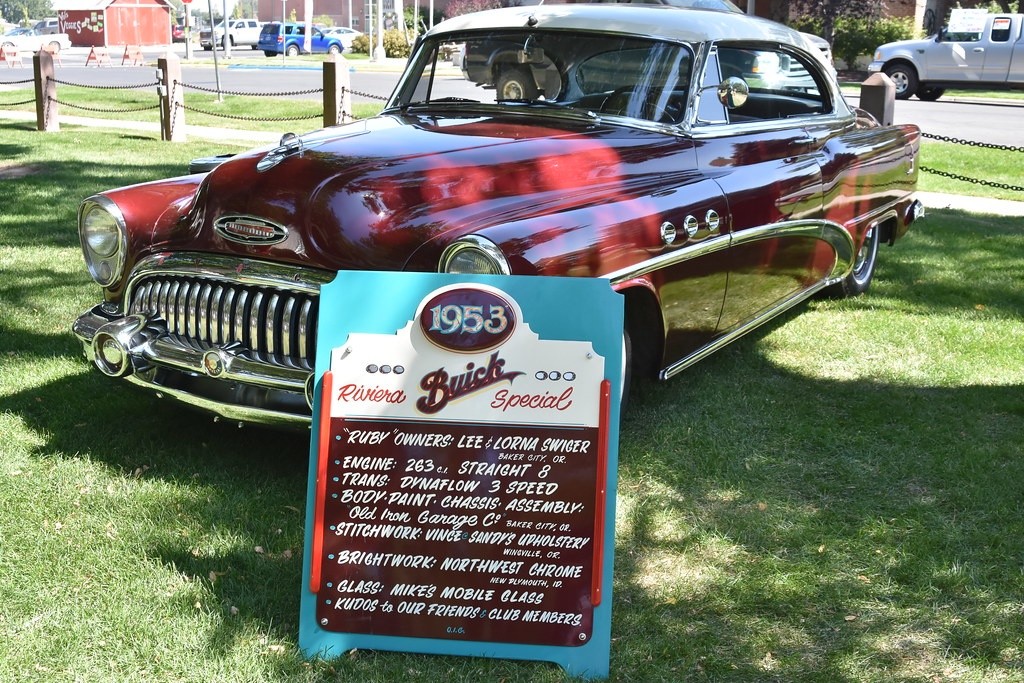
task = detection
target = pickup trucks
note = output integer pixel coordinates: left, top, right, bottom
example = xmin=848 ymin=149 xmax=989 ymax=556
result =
xmin=867 ymin=12 xmax=1024 ymax=102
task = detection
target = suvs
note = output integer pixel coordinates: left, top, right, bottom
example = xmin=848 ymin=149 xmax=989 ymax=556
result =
xmin=256 ymin=20 xmax=344 ymax=58
xmin=200 ymin=17 xmax=261 ymax=50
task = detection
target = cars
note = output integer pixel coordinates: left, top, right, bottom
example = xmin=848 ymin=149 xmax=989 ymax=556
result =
xmin=297 ymin=20 xmax=365 ymax=53
xmin=71 ymin=5 xmax=924 ymax=429
xmin=0 ymin=18 xmax=73 ymax=55
xmin=173 ymin=24 xmax=191 ymax=43
xmin=451 ymin=0 xmax=834 ymax=103
